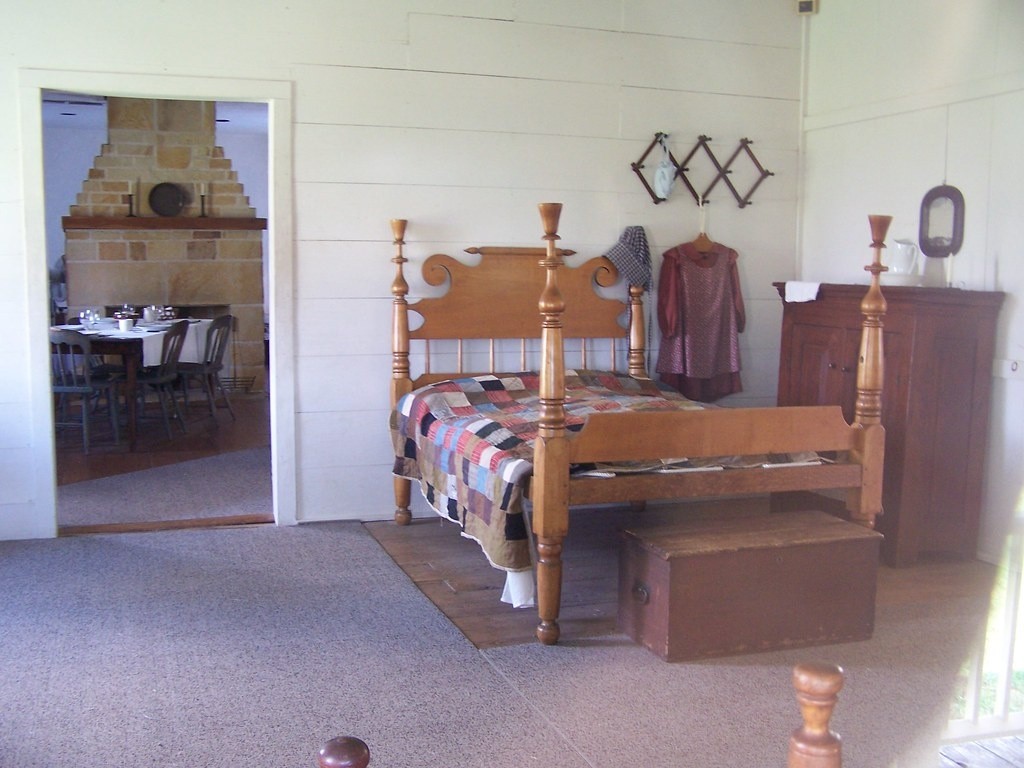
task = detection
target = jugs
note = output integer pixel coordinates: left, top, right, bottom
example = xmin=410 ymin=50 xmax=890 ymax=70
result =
xmin=891 ymin=240 xmax=920 ymax=275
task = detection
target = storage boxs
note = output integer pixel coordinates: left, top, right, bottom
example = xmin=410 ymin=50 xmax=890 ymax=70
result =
xmin=618 ymin=509 xmax=885 ymax=664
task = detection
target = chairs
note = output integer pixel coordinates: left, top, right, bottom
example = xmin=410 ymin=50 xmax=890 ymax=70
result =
xmin=48 ymin=315 xmax=235 ymax=455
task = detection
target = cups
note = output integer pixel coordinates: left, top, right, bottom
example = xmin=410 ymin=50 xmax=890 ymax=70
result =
xmin=122 ymin=303 xmax=135 ymax=313
xmin=119 ymin=319 xmax=133 ymax=331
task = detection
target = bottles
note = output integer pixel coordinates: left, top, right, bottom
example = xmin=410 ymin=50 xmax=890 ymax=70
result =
xmin=143 ymin=307 xmax=155 ymax=322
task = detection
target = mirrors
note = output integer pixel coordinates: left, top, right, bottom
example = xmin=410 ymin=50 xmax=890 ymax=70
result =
xmin=918 ymin=185 xmax=964 ymax=257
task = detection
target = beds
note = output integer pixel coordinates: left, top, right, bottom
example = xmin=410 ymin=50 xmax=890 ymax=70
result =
xmin=389 ymin=202 xmax=894 ymax=645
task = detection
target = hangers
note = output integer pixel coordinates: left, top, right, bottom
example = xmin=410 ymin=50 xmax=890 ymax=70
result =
xmin=693 ymin=233 xmax=715 ymax=254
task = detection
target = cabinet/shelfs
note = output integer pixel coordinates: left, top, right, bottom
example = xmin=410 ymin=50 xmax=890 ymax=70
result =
xmin=772 ymin=282 xmax=1004 ymax=567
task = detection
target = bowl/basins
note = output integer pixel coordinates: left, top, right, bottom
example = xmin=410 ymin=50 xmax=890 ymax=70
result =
xmin=880 ymin=274 xmax=922 ymax=287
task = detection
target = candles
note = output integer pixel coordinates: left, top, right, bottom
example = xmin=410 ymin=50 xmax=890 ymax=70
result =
xmin=128 ymin=180 xmax=132 ymax=194
xmin=201 ymin=183 xmax=204 ymax=194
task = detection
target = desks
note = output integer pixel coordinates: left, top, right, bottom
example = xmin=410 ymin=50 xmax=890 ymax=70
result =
xmin=49 ymin=328 xmax=169 ymax=453
xmin=99 ymin=317 xmax=215 ymax=422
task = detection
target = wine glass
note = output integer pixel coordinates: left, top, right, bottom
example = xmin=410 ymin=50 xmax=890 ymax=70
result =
xmin=79 ymin=308 xmax=101 ymax=332
